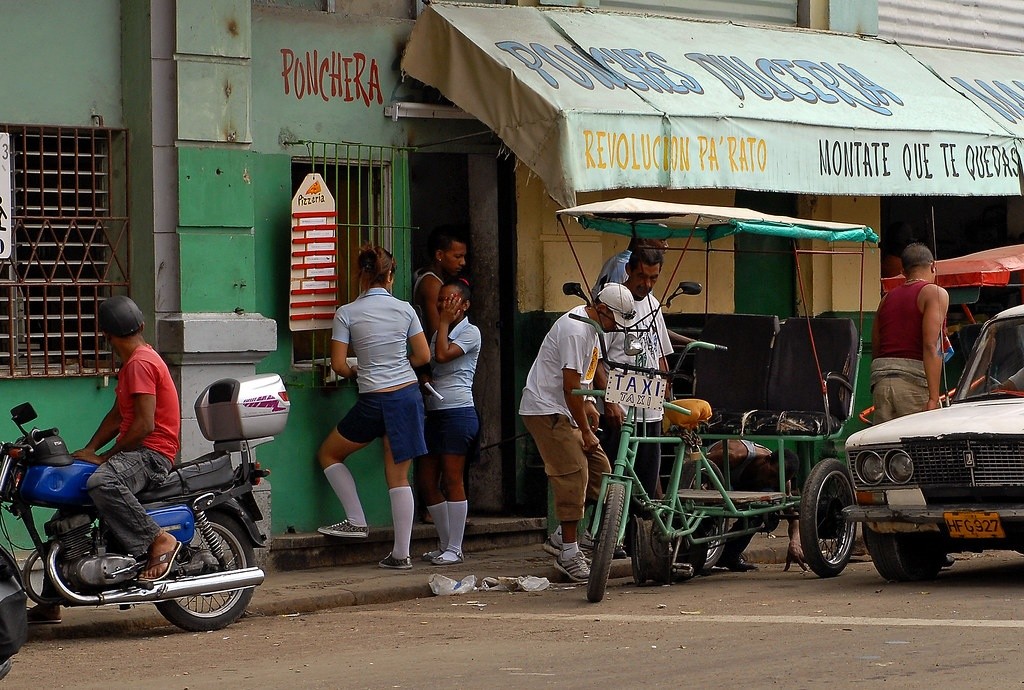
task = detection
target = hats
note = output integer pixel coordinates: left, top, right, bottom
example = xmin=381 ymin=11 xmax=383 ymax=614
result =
xmin=597 ymin=283 xmax=637 ymax=328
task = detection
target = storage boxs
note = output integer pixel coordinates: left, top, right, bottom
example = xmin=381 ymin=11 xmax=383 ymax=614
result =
xmin=193 ymin=372 xmax=292 ymax=442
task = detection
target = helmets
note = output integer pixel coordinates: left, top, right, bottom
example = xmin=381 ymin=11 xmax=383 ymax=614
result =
xmin=97 ymin=296 xmax=144 ymax=337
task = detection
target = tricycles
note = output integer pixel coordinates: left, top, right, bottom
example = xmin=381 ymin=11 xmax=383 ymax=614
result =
xmin=553 ymin=193 xmax=878 ymax=604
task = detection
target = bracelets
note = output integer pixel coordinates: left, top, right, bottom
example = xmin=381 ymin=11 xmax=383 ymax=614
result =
xmin=584 ymin=396 xmax=597 ymax=404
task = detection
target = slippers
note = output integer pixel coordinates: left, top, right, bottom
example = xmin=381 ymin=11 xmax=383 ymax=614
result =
xmin=25 ymin=605 xmax=61 ymax=624
xmin=143 ymin=541 xmax=182 ymax=581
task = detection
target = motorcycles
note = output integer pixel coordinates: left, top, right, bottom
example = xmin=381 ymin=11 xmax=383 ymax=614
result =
xmin=0 ymin=372 xmax=292 ymax=635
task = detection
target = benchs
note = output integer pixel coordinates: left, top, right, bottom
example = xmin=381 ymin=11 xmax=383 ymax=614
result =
xmin=680 ymin=311 xmax=859 ymax=438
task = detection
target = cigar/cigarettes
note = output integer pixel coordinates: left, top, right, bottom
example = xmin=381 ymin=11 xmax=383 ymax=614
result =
xmin=591 ymin=425 xmax=603 ymax=432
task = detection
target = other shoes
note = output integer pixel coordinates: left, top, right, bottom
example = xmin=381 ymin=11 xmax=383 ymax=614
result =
xmin=431 ymin=550 xmax=465 ymax=565
xmin=420 ymin=547 xmax=446 ymax=561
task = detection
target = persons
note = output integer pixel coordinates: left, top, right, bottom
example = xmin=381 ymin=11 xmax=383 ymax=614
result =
xmin=579 ymin=234 xmax=670 ymax=551
xmin=317 ymin=240 xmax=428 ymax=569
xmin=64 ymin=296 xmax=182 ymax=581
xmin=518 ymin=281 xmax=637 ymax=583
xmin=418 ymin=276 xmax=480 ymax=564
xmin=414 ymin=223 xmax=469 ymax=342
xmin=990 ymin=365 xmax=1024 ymax=392
xmin=595 ymin=244 xmax=677 ymax=558
xmin=869 ymin=243 xmax=950 ymax=427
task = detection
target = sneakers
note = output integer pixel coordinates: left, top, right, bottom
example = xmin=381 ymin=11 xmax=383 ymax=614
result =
xmin=317 ymin=520 xmax=369 ymax=539
xmin=554 ymin=550 xmax=589 ymax=581
xmin=543 ymin=532 xmax=598 ymax=556
xmin=377 ymin=552 xmax=413 ymax=569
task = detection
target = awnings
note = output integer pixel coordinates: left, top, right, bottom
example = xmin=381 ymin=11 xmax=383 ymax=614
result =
xmin=398 ymin=1 xmax=1024 ymax=206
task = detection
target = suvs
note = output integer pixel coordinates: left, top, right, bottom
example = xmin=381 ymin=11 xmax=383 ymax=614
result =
xmin=843 ymin=303 xmax=1024 ymax=585
